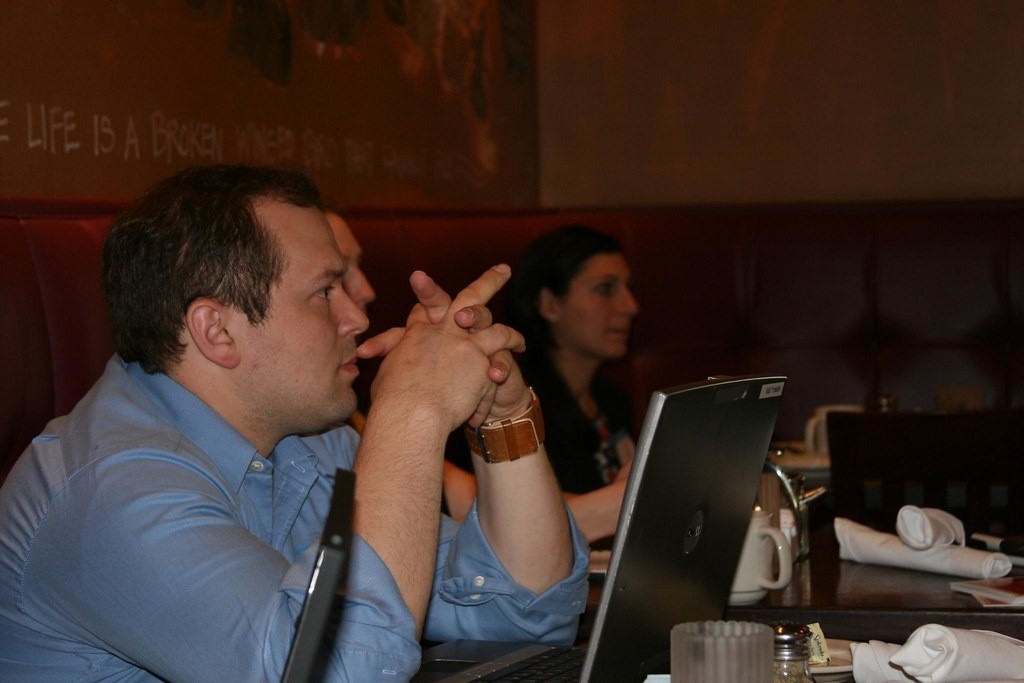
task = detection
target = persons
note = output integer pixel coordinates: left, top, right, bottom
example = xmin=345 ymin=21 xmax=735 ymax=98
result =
xmin=442 ymin=222 xmax=638 ymax=542
xmin=0 ymin=164 xmax=591 ymax=683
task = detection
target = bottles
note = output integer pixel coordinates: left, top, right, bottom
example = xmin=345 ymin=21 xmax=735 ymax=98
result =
xmin=769 ymin=620 xmax=813 ymax=683
xmin=777 ymin=472 xmax=811 ymax=558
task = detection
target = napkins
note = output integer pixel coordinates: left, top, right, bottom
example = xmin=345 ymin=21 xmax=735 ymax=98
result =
xmin=850 ymin=624 xmax=1024 ymax=683
xmin=834 ymin=515 xmax=1013 ymax=580
xmin=895 ymin=502 xmax=963 ymax=551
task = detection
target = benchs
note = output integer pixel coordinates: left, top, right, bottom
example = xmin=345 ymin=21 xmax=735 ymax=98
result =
xmin=0 ymin=194 xmax=1024 ymax=484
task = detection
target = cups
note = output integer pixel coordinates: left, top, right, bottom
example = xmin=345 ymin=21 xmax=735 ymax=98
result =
xmin=727 ymin=501 xmax=793 ymax=606
xmin=753 ymin=474 xmax=779 ymax=529
xmin=669 ymin=620 xmax=773 ymax=683
xmin=805 ymin=403 xmax=862 ymax=458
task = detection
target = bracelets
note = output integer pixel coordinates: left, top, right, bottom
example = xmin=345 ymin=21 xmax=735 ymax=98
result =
xmin=463 ymin=386 xmax=546 ymax=464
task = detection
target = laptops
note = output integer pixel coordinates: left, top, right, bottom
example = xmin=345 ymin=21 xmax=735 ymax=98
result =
xmin=403 ymin=373 xmax=790 ymax=683
xmin=281 ymin=466 xmax=357 ymax=683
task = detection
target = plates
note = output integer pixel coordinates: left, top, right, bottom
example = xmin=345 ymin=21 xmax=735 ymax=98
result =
xmin=807 ymin=638 xmax=854 ymax=673
